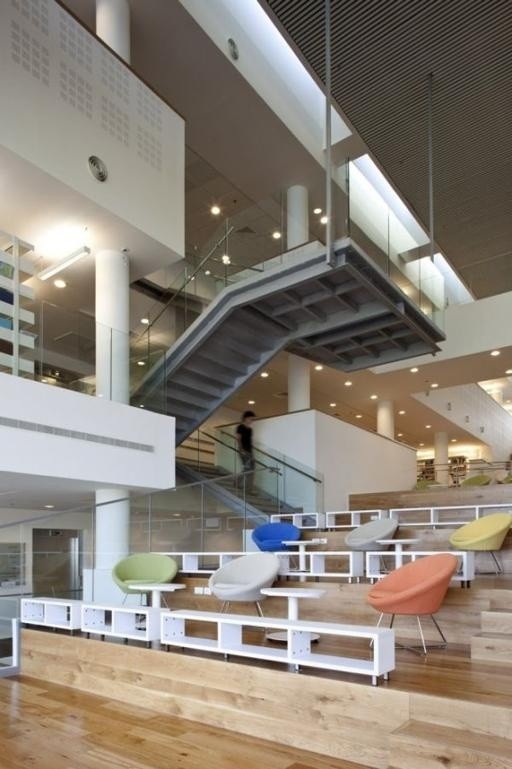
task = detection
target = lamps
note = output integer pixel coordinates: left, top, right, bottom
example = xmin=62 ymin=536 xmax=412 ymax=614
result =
xmin=37 ymin=246 xmax=90 ymax=282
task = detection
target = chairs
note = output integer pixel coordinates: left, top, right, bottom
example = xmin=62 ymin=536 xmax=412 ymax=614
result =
xmin=367 ymin=552 xmax=458 ymax=656
xmin=417 ymin=480 xmax=442 ymax=490
xmin=208 ymin=552 xmax=282 ymax=617
xmin=449 ymin=511 xmax=512 ymax=575
xmin=344 ymin=518 xmax=399 ymax=585
xmin=461 ymin=475 xmax=493 ymax=486
xmin=112 ymin=552 xmax=178 ymax=624
xmin=251 ymin=523 xmax=302 ymax=569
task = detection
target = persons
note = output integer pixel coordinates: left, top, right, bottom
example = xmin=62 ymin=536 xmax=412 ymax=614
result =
xmin=236 ymin=411 xmax=260 ymax=496
